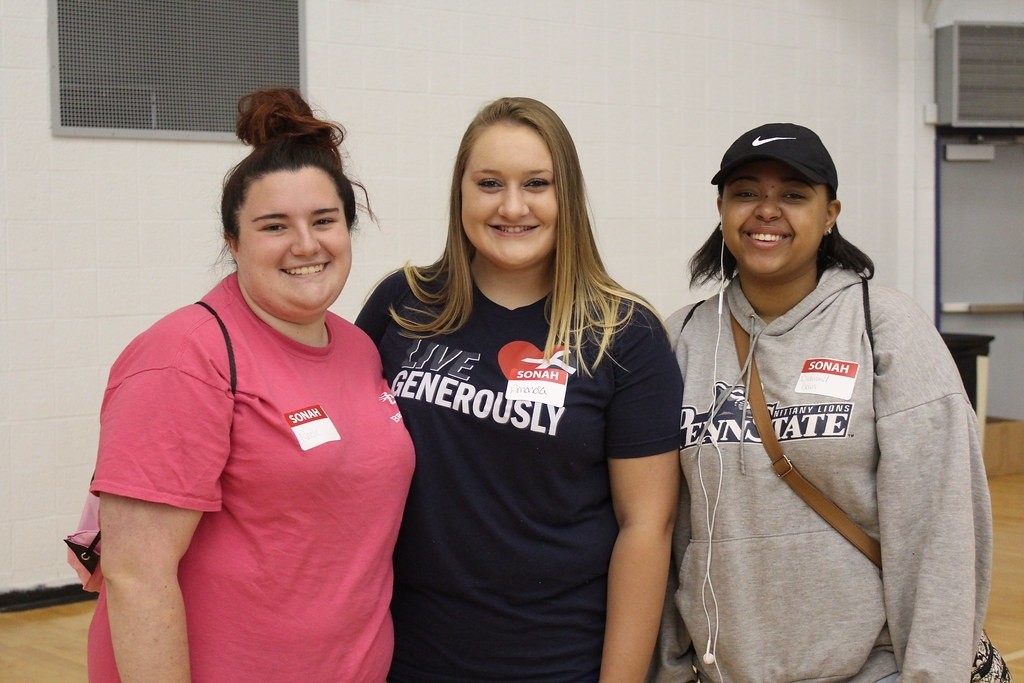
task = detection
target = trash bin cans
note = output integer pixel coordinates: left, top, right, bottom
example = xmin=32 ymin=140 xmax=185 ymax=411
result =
xmin=938 ymin=332 xmax=995 ymax=457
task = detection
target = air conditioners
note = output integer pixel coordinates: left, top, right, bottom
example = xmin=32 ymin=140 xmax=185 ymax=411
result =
xmin=935 ymin=20 xmax=1024 ymax=130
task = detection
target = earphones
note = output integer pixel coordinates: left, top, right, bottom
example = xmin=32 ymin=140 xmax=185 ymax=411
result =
xmin=703 ymin=640 xmax=715 ymax=664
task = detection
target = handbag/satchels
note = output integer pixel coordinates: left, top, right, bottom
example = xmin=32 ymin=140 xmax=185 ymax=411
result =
xmin=65 ymin=301 xmax=237 ymax=593
xmin=970 ymin=628 xmax=1013 ymax=683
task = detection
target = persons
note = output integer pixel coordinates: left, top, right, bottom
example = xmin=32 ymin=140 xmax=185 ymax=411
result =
xmin=661 ymin=123 xmax=993 ymax=683
xmin=87 ymin=87 xmax=416 ymax=683
xmin=352 ymin=95 xmax=683 ymax=683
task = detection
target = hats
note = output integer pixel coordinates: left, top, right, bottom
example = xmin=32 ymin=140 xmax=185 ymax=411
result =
xmin=711 ymin=123 xmax=838 ymax=193
